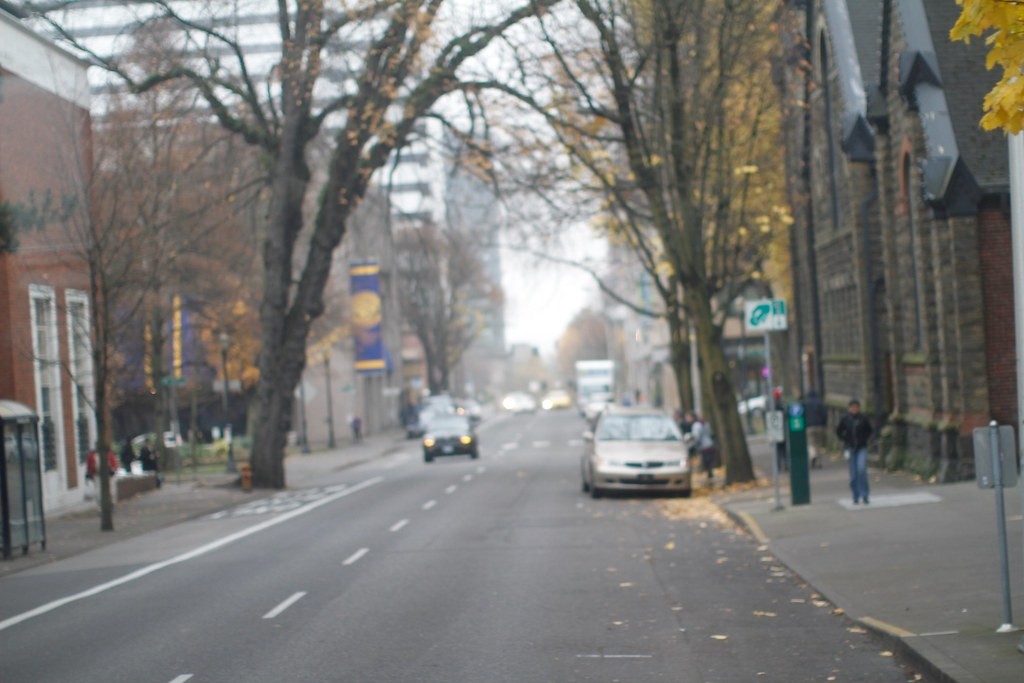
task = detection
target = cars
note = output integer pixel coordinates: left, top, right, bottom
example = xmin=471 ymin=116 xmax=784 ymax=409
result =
xmin=502 ymin=376 xmax=571 ymax=415
xmin=581 ymin=392 xmax=694 ymax=498
xmin=406 ymin=395 xmax=483 ymax=462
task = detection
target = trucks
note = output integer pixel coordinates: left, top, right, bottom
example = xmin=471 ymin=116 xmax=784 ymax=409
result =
xmin=574 ymin=358 xmax=615 ymax=417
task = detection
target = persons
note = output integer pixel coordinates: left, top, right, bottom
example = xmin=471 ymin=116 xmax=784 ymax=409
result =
xmin=835 ymin=400 xmax=874 ymax=505
xmin=684 ymin=411 xmax=721 ymax=479
xmin=351 ymin=414 xmax=364 ymax=443
xmin=85 ymin=442 xmax=117 ymax=513
xmin=803 ymin=388 xmax=828 ymax=469
xmin=119 ymin=437 xmax=136 ymax=472
xmin=774 ymin=389 xmax=788 ymax=473
xmin=139 ymin=439 xmax=162 ymax=489
